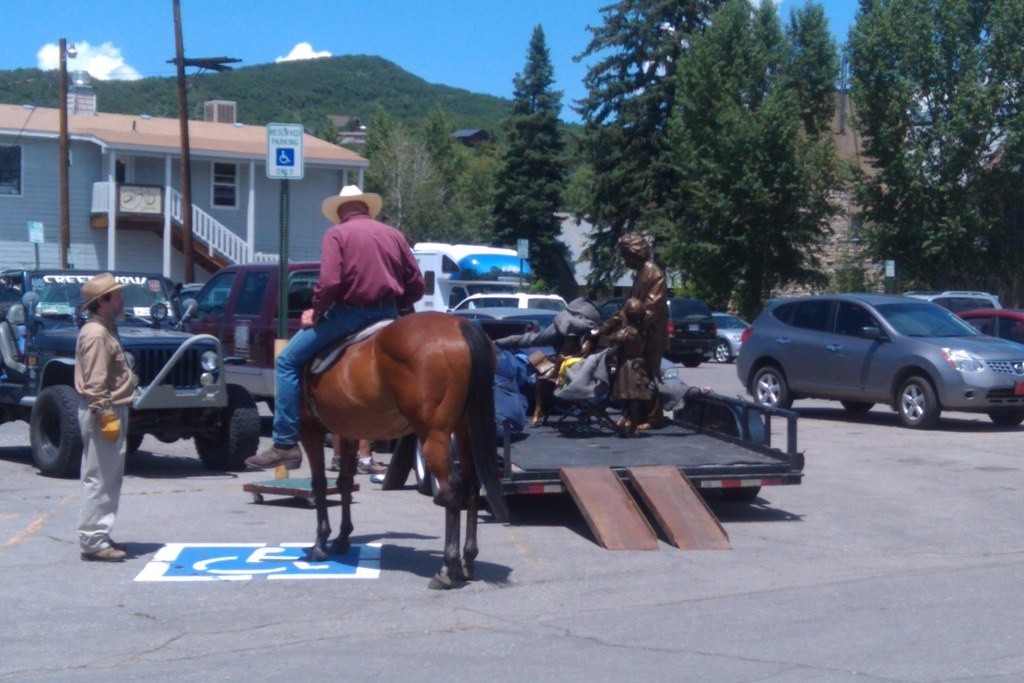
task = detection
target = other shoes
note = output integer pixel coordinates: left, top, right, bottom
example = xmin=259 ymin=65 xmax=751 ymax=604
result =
xmin=84 ymin=540 xmax=127 ymax=560
xmin=332 ymin=458 xmax=342 ymax=470
xmin=356 ymin=457 xmax=389 ymax=474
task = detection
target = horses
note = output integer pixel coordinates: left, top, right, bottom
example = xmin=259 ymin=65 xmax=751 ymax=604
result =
xmin=296 ymin=309 xmax=503 ymax=592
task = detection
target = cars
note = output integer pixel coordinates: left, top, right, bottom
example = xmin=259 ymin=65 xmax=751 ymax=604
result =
xmin=735 ymin=291 xmax=1023 ymax=431
xmin=950 ymin=308 xmax=1023 ymax=426
xmin=402 ymin=251 xmax=569 ymax=331
xmin=599 ymin=296 xmax=719 ymax=369
xmin=708 ymin=311 xmax=753 ymax=362
xmin=901 ymin=290 xmax=999 ymax=314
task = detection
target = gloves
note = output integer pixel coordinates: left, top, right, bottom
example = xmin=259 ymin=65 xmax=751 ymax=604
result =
xmin=101 ymin=414 xmax=121 ymax=444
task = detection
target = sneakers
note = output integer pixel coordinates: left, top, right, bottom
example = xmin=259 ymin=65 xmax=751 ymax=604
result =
xmin=245 ymin=442 xmax=303 ymax=470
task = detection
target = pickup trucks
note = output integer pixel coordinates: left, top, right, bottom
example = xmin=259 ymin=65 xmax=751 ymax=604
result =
xmin=181 ymin=262 xmax=543 ymax=430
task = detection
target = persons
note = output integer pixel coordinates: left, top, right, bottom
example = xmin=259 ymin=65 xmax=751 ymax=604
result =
xmin=245 ymin=186 xmax=425 ymax=470
xmin=331 ymin=433 xmax=390 ymax=474
xmin=73 ymin=273 xmax=140 ymax=561
xmin=608 ymin=297 xmax=654 ymax=436
xmin=597 ymin=231 xmax=670 ymax=430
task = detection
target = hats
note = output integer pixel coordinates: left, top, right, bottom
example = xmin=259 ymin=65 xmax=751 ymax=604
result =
xmin=322 ymin=184 xmax=384 ymax=224
xmin=80 ymin=271 xmax=129 ymax=312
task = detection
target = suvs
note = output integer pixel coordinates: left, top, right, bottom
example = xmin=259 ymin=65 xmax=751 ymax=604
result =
xmin=0 ymin=264 xmax=265 ymax=483
